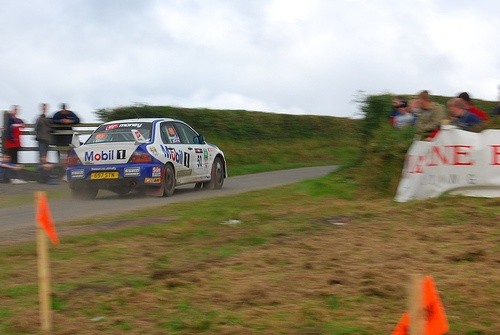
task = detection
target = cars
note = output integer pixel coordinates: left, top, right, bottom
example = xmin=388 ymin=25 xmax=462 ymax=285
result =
xmin=66 ymin=118 xmax=228 ymax=198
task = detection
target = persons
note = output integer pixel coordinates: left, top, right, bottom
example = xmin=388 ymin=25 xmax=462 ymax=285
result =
xmin=387 ymin=90 xmax=490 ymax=144
xmin=0 ymin=103 xmax=79 ymax=184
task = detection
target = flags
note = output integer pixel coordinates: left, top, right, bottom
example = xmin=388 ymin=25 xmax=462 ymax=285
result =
xmin=35 ymin=194 xmax=62 ymax=246
xmin=390 ymin=276 xmax=450 ymax=335
